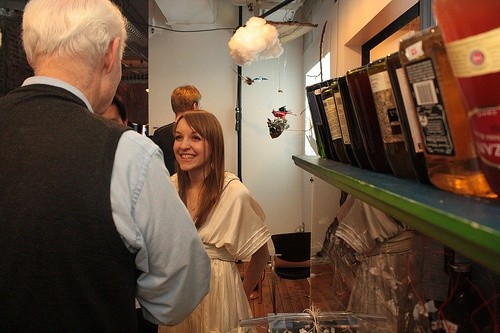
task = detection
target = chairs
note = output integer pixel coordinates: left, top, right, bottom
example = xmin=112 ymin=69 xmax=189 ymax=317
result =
xmin=269 ymin=231 xmax=311 ymax=315
xmin=233 ymin=253 xmax=265 ymax=304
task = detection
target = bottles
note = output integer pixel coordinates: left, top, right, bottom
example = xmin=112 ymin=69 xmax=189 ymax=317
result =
xmin=440 ymin=260 xmax=489 ymax=333
xmin=305 ymin=0 xmax=500 ymax=204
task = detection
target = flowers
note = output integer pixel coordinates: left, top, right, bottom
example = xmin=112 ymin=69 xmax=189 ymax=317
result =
xmin=266 ymin=106 xmax=298 ymax=140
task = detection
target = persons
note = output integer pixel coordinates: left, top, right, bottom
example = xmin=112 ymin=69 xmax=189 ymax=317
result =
xmin=100 ymin=96 xmax=136 ymax=130
xmin=0 ymin=0 xmax=212 ymax=333
xmin=156 ymin=108 xmax=272 ymax=333
xmin=152 ymin=85 xmax=203 ymax=177
